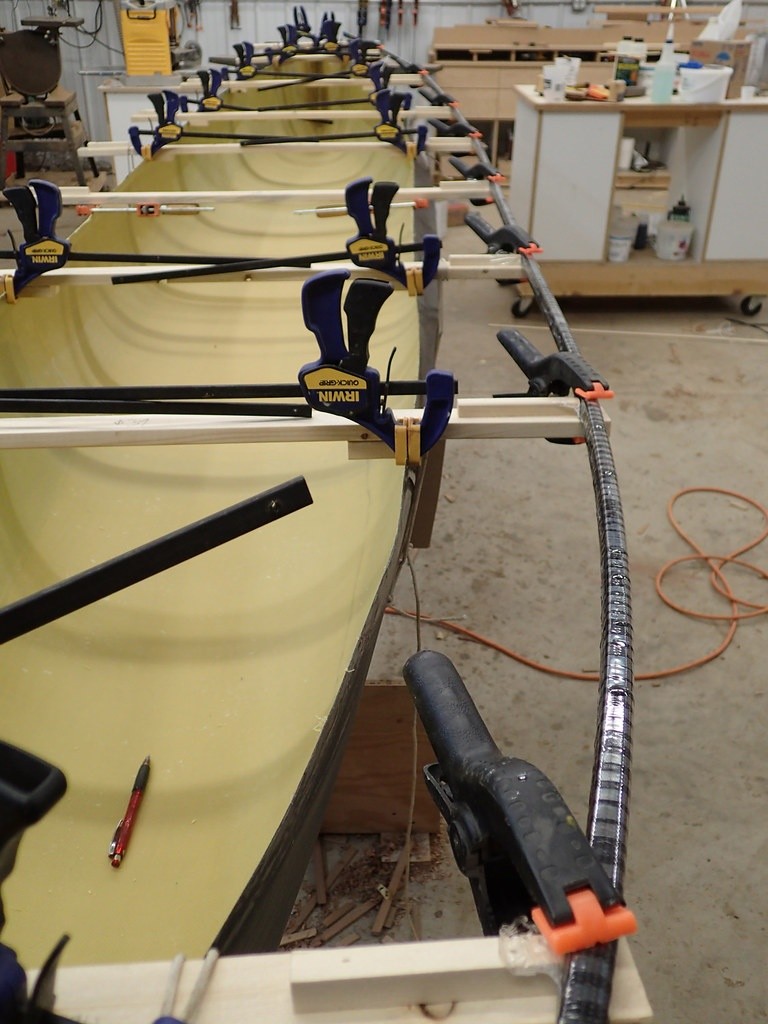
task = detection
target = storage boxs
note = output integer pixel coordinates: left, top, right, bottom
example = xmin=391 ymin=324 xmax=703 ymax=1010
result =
xmin=687 ymin=38 xmax=749 ymax=98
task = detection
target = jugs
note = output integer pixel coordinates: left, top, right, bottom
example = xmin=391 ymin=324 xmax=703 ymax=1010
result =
xmin=667 ymin=205 xmax=691 ymax=222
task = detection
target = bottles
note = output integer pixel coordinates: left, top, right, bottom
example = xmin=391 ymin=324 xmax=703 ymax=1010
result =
xmin=612 ymin=36 xmax=675 ymax=103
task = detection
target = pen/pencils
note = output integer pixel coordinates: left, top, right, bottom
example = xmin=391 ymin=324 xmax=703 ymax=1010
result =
xmin=107 ymin=754 xmax=151 ymax=868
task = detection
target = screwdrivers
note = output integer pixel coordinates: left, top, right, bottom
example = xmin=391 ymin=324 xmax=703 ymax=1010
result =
xmin=353 ymin=1 xmax=394 ymax=44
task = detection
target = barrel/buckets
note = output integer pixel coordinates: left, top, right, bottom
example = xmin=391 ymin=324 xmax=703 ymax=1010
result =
xmin=677 ymin=63 xmax=733 ymax=102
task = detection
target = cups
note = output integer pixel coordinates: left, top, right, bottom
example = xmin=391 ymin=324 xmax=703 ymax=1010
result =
xmin=541 ymin=56 xmax=582 ymax=102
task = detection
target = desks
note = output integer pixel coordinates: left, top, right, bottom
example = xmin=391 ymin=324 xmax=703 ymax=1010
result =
xmin=98 ymin=79 xmax=212 ymax=183
xmin=503 ymin=87 xmax=765 ymax=324
